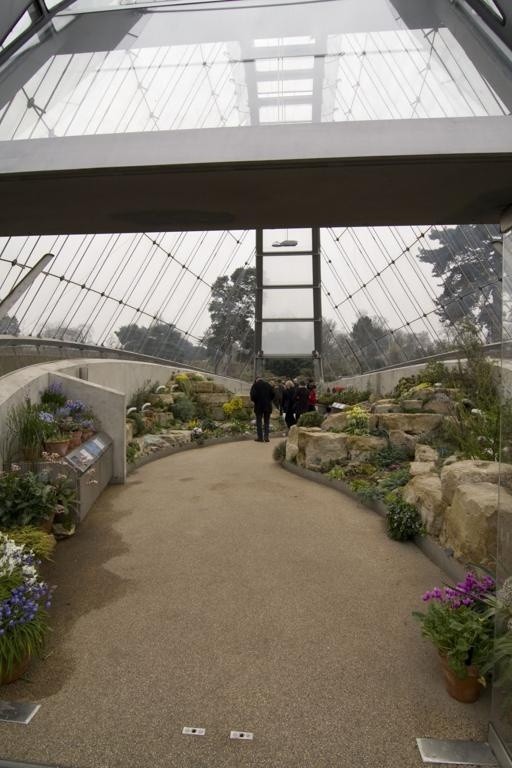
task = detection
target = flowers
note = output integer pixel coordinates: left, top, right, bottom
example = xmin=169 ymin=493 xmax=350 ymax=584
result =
xmin=0 ymin=457 xmax=95 ymax=680
xmin=412 ymin=571 xmax=496 ymax=679
xmin=2 ymin=383 xmax=98 ymax=449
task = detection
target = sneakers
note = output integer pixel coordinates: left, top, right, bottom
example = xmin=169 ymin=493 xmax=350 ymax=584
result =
xmin=254 ymin=438 xmax=269 ymax=442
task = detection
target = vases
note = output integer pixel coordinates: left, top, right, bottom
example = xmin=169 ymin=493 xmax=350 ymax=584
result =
xmin=441 ymin=658 xmax=483 ymax=704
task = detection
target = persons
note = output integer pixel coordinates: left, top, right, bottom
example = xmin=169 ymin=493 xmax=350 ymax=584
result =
xmin=250 ymin=373 xmax=276 ymax=442
xmin=269 ymin=377 xmax=318 ymax=430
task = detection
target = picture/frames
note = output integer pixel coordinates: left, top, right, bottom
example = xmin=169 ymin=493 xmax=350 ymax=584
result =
xmin=47 ymin=432 xmax=92 ymax=456
